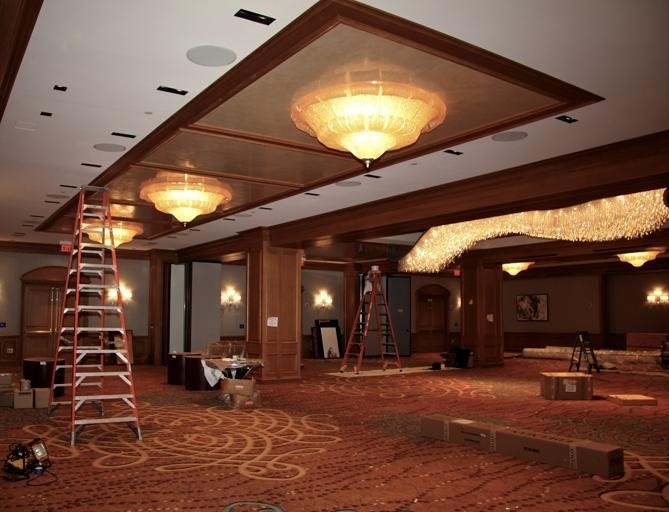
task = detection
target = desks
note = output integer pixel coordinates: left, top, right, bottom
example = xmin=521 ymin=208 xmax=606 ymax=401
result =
xmin=184 ymin=356 xmax=264 ymax=391
xmin=167 ymin=352 xmax=205 ymax=385
xmin=24 ymin=357 xmax=65 ymax=398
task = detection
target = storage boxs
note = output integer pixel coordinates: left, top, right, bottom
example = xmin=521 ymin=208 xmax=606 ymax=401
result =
xmin=14 ymin=388 xmax=34 ymax=409
xmin=0 ymin=382 xmax=20 ymax=407
xmin=0 ymin=373 xmax=13 ymax=384
xmin=540 ymin=372 xmax=594 ymax=401
xmin=220 ymin=378 xmax=254 ymax=397
xmin=231 ymin=391 xmax=262 ymax=408
xmin=34 ymin=387 xmax=50 ymax=408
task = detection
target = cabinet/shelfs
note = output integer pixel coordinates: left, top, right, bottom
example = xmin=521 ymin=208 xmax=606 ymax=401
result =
xmin=625 ymin=332 xmax=669 ymax=351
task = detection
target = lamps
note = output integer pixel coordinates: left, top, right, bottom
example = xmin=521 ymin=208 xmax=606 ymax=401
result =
xmin=139 ymin=176 xmax=232 ymax=229
xmin=502 ymin=262 xmax=535 ymax=276
xmin=616 ymin=251 xmax=660 ymax=267
xmin=80 ymin=217 xmax=144 ymax=249
xmin=290 ymin=81 xmax=448 ymax=168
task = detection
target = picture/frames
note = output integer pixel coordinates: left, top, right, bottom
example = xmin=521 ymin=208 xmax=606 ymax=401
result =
xmin=516 ymin=293 xmax=549 ymax=322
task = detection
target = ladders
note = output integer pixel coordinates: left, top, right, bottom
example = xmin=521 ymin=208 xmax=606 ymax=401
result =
xmin=339 ymin=269 xmax=403 ymax=373
xmin=47 ymin=184 xmax=142 ymax=448
